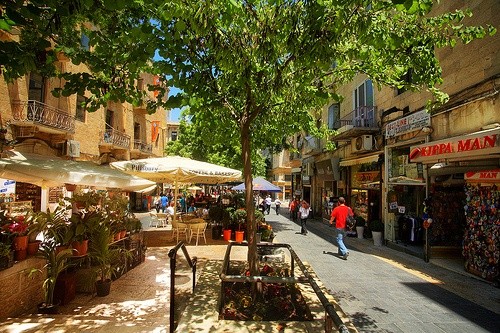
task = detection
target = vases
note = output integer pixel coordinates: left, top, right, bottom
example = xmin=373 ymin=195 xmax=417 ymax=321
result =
xmin=12 ymin=235 xmax=28 ymax=251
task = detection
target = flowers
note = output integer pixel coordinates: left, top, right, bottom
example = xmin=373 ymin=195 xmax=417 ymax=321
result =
xmin=3 ymin=215 xmax=28 ymax=236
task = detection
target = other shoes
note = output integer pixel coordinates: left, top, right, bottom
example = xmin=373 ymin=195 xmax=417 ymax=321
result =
xmin=344 ymin=252 xmax=349 ymax=260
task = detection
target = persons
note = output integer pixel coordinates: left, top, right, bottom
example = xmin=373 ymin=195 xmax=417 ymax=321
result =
xmin=297 ymin=199 xmax=312 ymax=236
xmin=261 ymin=197 xmax=269 ymax=214
xmin=176 ymin=192 xmax=204 ymax=213
xmin=9 ymin=193 xmax=15 ymax=202
xmin=63 ymin=182 xmax=82 ymax=215
xmin=0 ymin=193 xmax=5 ymax=202
xmin=288 ymin=196 xmax=300 ymax=221
xmin=153 ymin=193 xmax=174 ymax=215
xmin=204 ymin=186 xmax=234 ymax=201
xmin=274 ymin=197 xmax=281 ymax=215
xmin=265 ymin=195 xmax=271 ymax=214
xmin=329 ymin=197 xmax=354 ymax=261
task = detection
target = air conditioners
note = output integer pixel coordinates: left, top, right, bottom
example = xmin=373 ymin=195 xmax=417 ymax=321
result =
xmin=351 ymin=135 xmax=372 ymax=153
xmin=63 ymin=140 xmax=80 ymax=157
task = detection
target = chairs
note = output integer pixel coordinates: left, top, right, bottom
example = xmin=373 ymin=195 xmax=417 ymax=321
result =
xmin=149 ymin=212 xmax=208 ymax=246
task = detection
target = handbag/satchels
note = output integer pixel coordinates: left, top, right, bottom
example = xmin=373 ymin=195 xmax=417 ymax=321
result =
xmin=346 ymin=215 xmax=357 ymax=231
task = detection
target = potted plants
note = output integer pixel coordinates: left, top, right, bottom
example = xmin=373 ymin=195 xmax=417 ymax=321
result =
xmin=372 ymin=221 xmax=384 ymax=247
xmin=208 ymin=200 xmax=275 ymax=244
xmin=0 ymin=190 xmax=145 ymax=314
xmin=355 ymin=216 xmax=365 ymax=238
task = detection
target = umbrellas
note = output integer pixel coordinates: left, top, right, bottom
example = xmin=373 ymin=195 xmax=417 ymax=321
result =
xmin=110 ymin=156 xmax=242 ymax=220
xmin=0 ymin=155 xmax=157 ymax=196
xmin=165 ymin=182 xmax=202 ymax=191
xmin=229 ymin=176 xmax=282 ymax=211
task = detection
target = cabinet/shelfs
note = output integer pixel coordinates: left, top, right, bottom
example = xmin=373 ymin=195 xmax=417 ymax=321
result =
xmin=49 ymin=189 xmax=61 ymax=202
xmin=0 ymin=193 xmax=15 ymax=212
xmin=67 ymin=235 xmax=145 ymax=292
xmin=1 ymin=200 xmax=33 ymax=214
xmin=15 ymin=185 xmax=40 ymax=213
